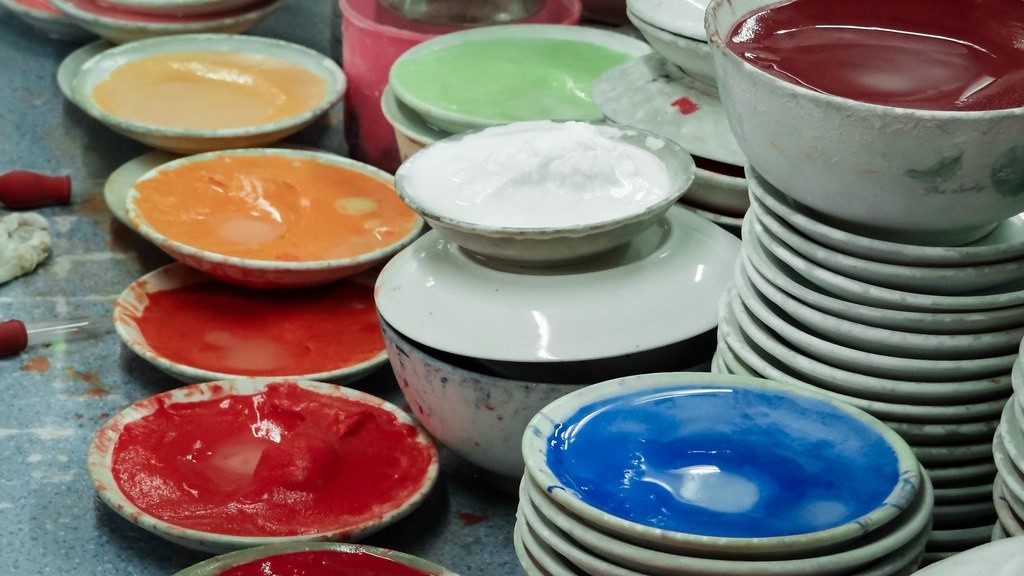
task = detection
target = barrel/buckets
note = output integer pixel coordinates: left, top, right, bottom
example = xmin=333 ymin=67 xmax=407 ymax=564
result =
xmin=339 ymin=0 xmax=586 ymax=164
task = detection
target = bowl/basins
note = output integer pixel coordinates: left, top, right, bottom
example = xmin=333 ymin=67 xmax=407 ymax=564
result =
xmin=113 ymin=260 xmax=394 ymax=386
xmin=513 ymin=373 xmax=937 ymax=576
xmin=393 ymin=120 xmax=698 ymax=263
xmin=133 ymin=150 xmax=422 ymax=289
xmin=87 ymin=379 xmax=442 ymax=545
xmin=385 ymin=20 xmax=657 ymax=165
xmin=703 ymin=0 xmax=1024 ymax=240
xmin=72 ymin=29 xmax=347 ymax=156
xmin=709 ymin=165 xmax=1024 ymax=565
xmin=374 ymin=299 xmax=723 ymax=480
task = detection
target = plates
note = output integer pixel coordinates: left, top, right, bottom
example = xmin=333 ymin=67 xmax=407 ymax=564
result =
xmin=593 ymin=54 xmax=750 ymax=170
xmin=375 ymin=208 xmax=750 ymax=361
